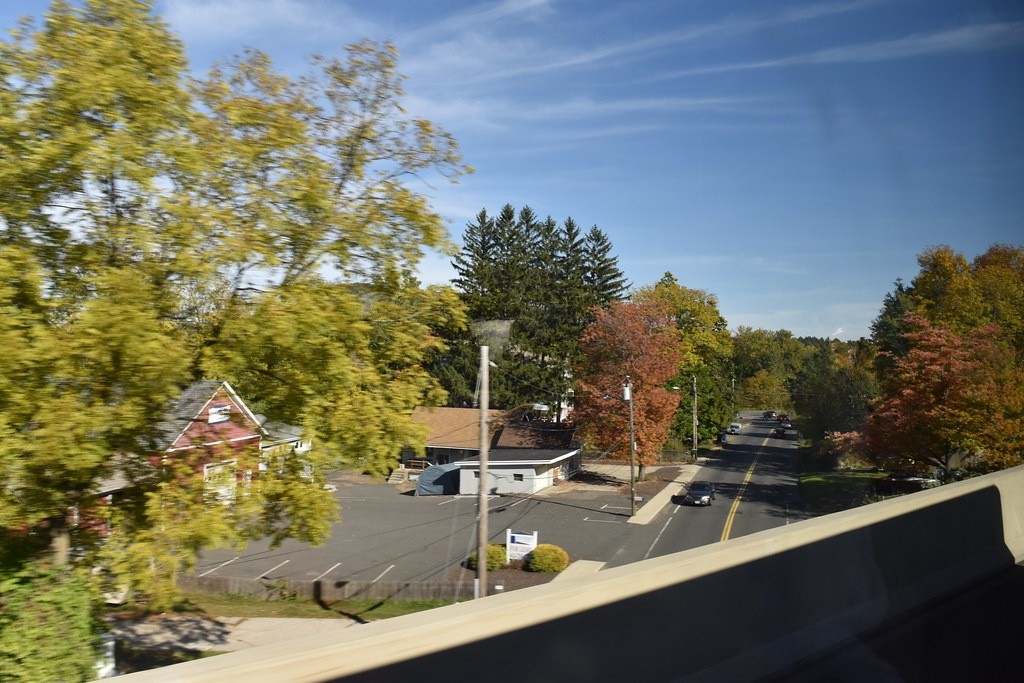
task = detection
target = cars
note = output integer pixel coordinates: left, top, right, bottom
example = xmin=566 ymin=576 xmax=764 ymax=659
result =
xmin=728 ymin=422 xmax=742 ymax=434
xmin=774 ymin=428 xmax=786 ymax=438
xmin=685 ymin=481 xmax=716 ymax=506
xmin=763 ymin=411 xmax=792 ymax=429
